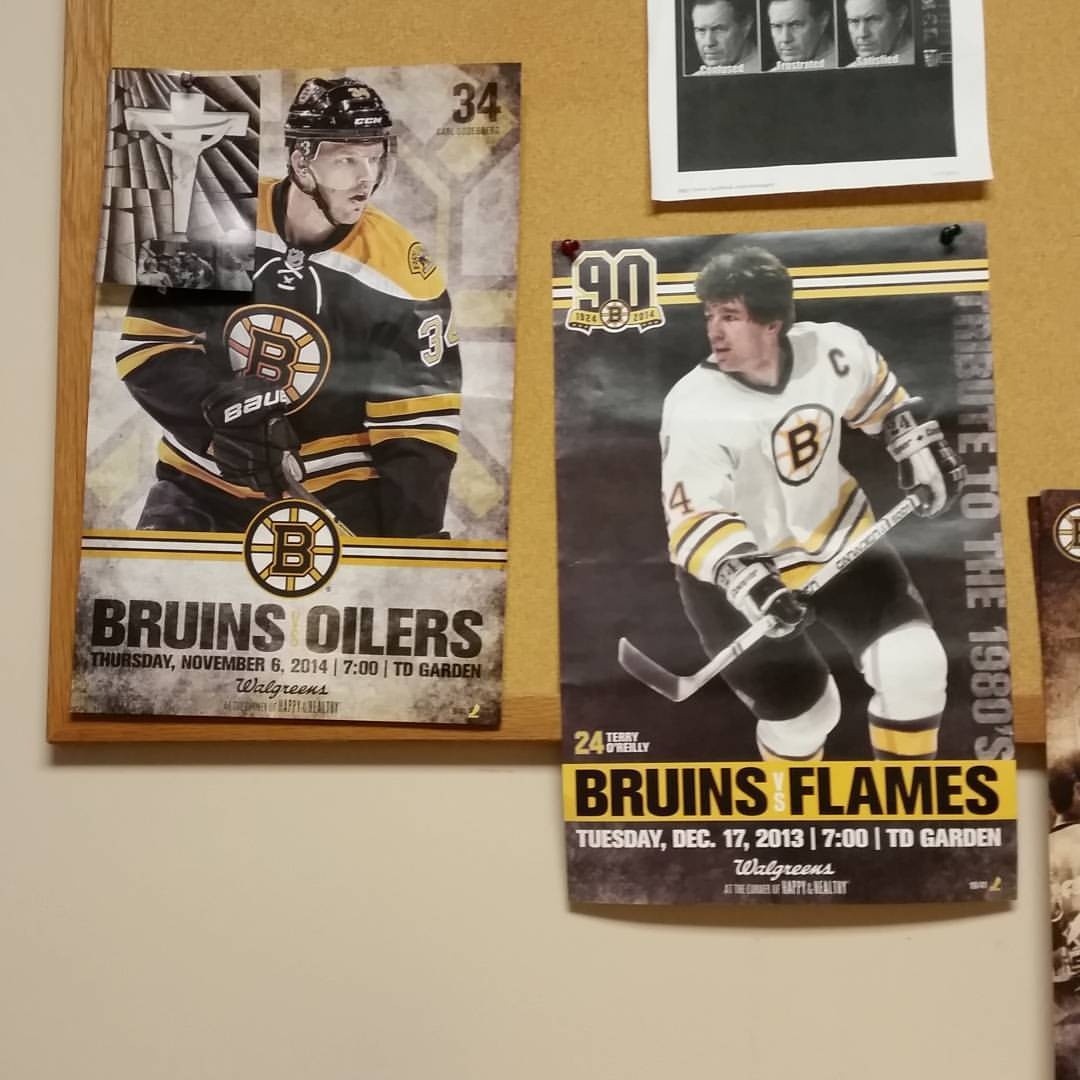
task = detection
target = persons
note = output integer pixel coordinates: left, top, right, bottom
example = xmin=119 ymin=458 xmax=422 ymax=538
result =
xmin=844 ymin=0 xmax=914 ymax=66
xmin=690 ymin=0 xmax=758 ymax=73
xmin=115 ymin=77 xmax=464 ymax=538
xmin=765 ymin=0 xmax=835 ymax=69
xmin=1048 ymin=748 xmax=1080 ymax=1080
xmin=660 ymin=244 xmax=969 ymax=758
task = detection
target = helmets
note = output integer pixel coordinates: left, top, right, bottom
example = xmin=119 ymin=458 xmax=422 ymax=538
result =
xmin=283 ymin=76 xmax=394 ymax=162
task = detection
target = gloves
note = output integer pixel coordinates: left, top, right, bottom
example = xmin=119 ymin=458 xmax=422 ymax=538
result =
xmin=200 ymin=379 xmax=308 ymax=506
xmin=883 ymin=395 xmax=968 ymax=521
xmin=712 ymin=551 xmax=806 ymax=639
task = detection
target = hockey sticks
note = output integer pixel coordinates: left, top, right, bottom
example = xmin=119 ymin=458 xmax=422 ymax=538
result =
xmin=616 ymin=485 xmax=934 ymax=704
xmin=280 ymin=474 xmax=354 ymax=535
